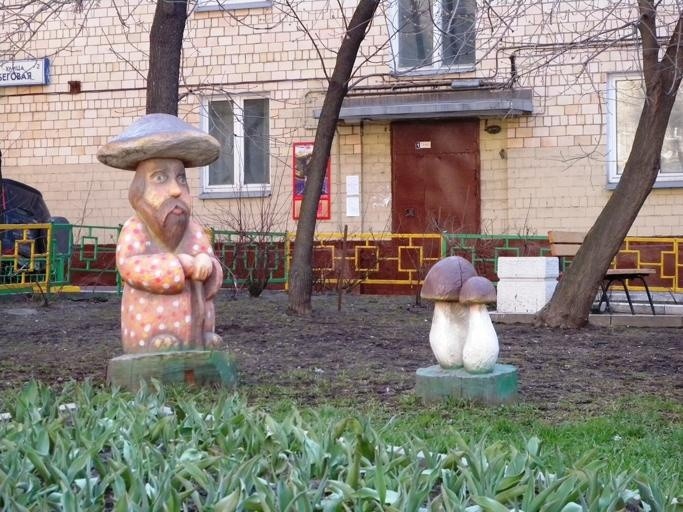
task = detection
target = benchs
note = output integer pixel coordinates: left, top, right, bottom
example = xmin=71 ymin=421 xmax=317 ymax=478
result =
xmin=547 ymin=230 xmax=655 ymax=314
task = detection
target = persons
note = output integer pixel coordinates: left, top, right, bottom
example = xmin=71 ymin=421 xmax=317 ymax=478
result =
xmin=116 ymin=158 xmax=227 ymax=353
